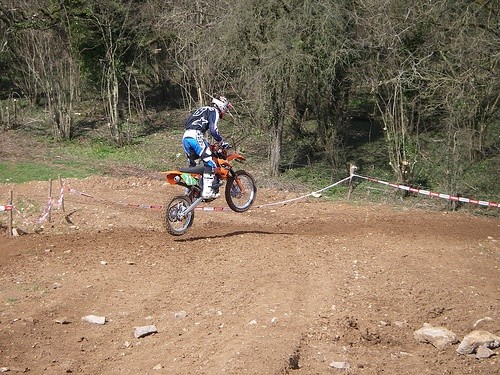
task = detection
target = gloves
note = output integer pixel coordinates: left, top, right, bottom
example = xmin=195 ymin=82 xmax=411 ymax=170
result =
xmin=219 ymin=139 xmax=230 ymax=150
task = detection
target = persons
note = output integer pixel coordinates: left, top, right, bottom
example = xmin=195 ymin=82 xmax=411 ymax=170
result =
xmin=182 ymin=93 xmax=232 ymax=201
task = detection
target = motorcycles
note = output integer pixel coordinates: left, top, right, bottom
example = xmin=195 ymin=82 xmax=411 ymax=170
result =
xmin=159 ymin=143 xmax=256 ymax=236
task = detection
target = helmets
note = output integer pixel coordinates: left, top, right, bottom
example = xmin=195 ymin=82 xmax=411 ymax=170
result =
xmin=211 ymin=94 xmax=229 ymax=119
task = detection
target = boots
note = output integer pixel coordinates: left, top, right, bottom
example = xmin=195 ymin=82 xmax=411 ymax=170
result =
xmin=201 ymin=173 xmax=220 ymax=199
